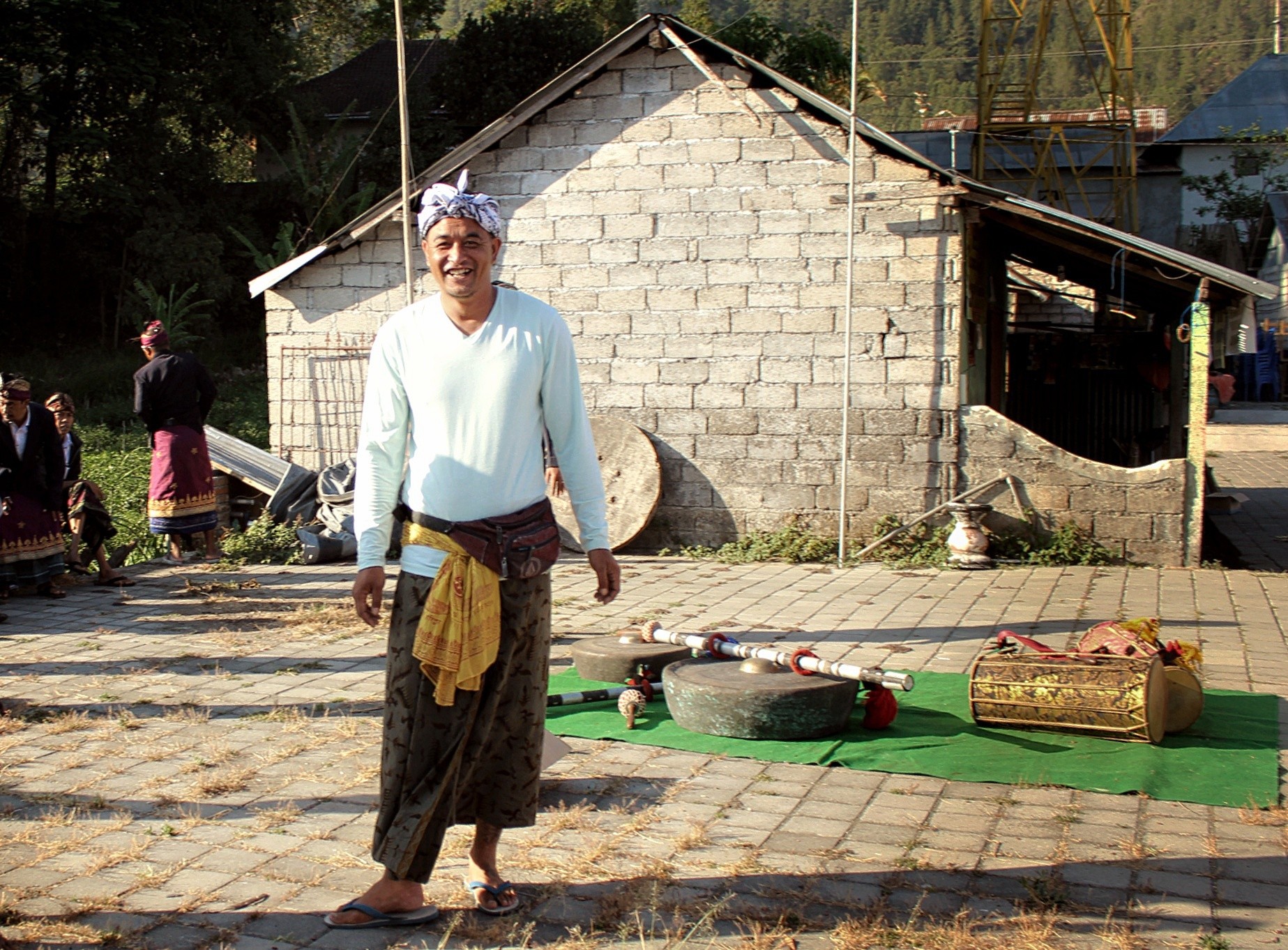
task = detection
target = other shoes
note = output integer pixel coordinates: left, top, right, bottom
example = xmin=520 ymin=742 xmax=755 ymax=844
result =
xmin=0 ymin=613 xmax=8 ymax=623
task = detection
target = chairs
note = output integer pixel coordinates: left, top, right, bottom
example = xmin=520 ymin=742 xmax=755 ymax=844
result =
xmin=1244 ymin=330 xmax=1279 ymax=402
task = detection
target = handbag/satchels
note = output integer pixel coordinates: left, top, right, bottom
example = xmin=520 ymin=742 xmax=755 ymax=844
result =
xmin=392 ymin=479 xmax=561 ymax=581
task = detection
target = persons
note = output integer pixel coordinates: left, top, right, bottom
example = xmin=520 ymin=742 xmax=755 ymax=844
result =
xmin=134 ymin=320 xmax=222 ymax=566
xmin=0 ymin=372 xmax=136 ymax=600
xmin=323 ymin=169 xmax=621 ymax=932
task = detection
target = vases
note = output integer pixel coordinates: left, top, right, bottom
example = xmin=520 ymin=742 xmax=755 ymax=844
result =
xmin=946 ymin=502 xmax=993 ymax=568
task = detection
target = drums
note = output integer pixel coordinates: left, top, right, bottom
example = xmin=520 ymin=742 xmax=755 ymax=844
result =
xmin=966 ymin=644 xmax=1169 ymax=748
xmin=1072 ymin=621 xmax=1199 ymax=731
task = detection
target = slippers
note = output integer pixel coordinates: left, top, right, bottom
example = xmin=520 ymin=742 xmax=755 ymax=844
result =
xmin=323 ymin=897 xmax=439 ymax=929
xmin=94 ymin=576 xmax=136 ymax=587
xmin=63 ymin=561 xmax=92 ymax=576
xmin=37 ymin=584 xmax=66 ymax=598
xmin=162 ymin=552 xmax=182 ymax=565
xmin=464 ymin=876 xmax=519 ymax=913
xmin=0 ymin=584 xmax=11 ymax=599
xmin=205 ymin=550 xmax=231 ymax=563
